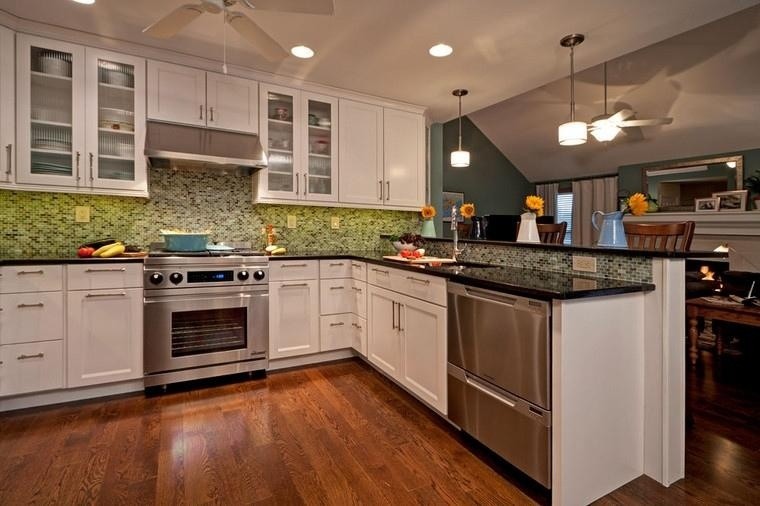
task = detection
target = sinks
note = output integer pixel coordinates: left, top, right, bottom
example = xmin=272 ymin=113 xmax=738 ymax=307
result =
xmin=411 ymin=258 xmax=504 ymax=269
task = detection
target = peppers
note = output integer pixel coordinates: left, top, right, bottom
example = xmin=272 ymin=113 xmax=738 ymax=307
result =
xmin=91 ymin=241 xmax=126 ymax=258
xmin=271 ymin=247 xmax=286 ymax=254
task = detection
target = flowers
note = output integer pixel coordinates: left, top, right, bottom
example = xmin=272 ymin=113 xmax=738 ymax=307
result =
xmin=458 ymin=202 xmax=477 ymax=219
xmin=520 ymin=193 xmax=545 ymax=218
xmin=421 ymin=203 xmax=437 ymax=219
xmin=619 ymin=192 xmax=650 ymax=218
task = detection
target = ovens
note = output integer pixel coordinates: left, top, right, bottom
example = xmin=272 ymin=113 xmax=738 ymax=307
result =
xmin=141 ymin=286 xmax=270 ymax=395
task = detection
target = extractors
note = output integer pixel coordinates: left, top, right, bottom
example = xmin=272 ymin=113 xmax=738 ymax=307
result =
xmin=143 ymin=121 xmax=269 ymax=178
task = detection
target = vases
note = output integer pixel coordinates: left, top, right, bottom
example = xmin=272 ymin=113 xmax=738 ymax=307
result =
xmin=420 ymin=216 xmax=438 ymax=240
xmin=468 ymin=215 xmax=488 ymax=242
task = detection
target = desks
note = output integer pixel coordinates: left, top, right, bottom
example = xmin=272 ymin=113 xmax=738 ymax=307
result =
xmin=685 ymin=293 xmax=760 ymax=366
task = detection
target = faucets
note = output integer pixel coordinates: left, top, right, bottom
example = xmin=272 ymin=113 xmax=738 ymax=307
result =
xmin=449 ymin=204 xmax=460 ymax=257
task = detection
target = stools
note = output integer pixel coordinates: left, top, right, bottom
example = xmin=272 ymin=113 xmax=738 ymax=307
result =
xmin=685 ymin=279 xmax=717 ymax=331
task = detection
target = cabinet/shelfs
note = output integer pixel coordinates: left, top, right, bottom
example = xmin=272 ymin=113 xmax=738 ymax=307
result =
xmin=0 ymin=23 xmax=17 ymax=192
xmin=0 ymin=265 xmax=65 ymax=400
xmin=16 ymin=29 xmax=149 ymax=199
xmin=147 ymin=54 xmax=262 ymax=139
xmin=65 ymin=265 xmax=145 ymax=393
xmin=265 ymin=261 xmax=321 ymax=363
xmin=338 ymin=93 xmax=428 ymax=209
xmin=368 ymin=263 xmax=446 ymax=419
xmin=320 ymin=259 xmax=350 ymax=359
xmin=350 ymin=262 xmax=368 ymax=360
xmin=250 ymin=80 xmax=339 ymax=206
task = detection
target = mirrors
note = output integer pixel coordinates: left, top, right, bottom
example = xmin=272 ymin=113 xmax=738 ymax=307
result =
xmin=639 ymin=154 xmax=746 ymax=212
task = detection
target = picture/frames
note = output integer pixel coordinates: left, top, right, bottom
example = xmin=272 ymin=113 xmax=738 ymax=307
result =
xmin=710 ymin=189 xmax=750 ymax=214
xmin=692 ymin=196 xmax=722 ymax=213
xmin=442 ymin=190 xmax=466 ymax=224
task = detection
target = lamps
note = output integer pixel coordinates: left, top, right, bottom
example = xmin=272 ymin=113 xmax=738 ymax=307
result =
xmin=556 ymin=30 xmax=590 ymax=149
xmin=589 ymin=114 xmax=623 ymax=149
xmin=449 ymin=83 xmax=472 ymax=171
xmin=712 ymin=239 xmax=760 ymax=273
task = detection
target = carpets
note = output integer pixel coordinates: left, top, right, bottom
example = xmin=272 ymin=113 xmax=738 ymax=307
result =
xmin=687 ymin=320 xmax=746 ymax=361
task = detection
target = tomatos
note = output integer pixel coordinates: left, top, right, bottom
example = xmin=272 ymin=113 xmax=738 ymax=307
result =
xmin=400 ymin=250 xmax=421 ymax=257
xmin=78 ymin=248 xmax=95 ymax=258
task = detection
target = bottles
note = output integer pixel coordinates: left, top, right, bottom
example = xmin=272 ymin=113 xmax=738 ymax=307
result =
xmin=264 ymin=108 xmax=330 ymax=193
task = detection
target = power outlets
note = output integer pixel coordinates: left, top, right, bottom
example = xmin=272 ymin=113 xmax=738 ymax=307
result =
xmin=568 ymin=252 xmax=601 ymax=277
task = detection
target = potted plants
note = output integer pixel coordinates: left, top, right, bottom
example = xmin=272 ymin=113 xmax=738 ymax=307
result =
xmin=743 ymin=169 xmax=760 ymax=210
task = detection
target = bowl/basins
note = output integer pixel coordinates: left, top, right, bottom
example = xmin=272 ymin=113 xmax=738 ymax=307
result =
xmin=37 ymin=54 xmax=71 ymax=76
xmin=102 ymin=69 xmax=134 ymax=86
xmin=160 ymin=232 xmax=219 ymax=252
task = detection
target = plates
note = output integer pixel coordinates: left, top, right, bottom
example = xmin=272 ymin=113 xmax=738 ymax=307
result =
xmin=31 ymin=107 xmax=133 ymax=182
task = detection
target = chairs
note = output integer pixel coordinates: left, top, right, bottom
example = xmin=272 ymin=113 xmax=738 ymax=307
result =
xmin=711 ymin=271 xmax=759 ymax=339
xmin=515 ymin=220 xmax=568 ymax=244
xmin=622 ymin=219 xmax=697 ymax=251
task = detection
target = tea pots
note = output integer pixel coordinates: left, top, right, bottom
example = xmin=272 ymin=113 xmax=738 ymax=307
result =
xmin=469 ymin=216 xmax=493 ymax=239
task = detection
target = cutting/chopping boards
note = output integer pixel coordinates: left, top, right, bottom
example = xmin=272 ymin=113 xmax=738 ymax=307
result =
xmin=382 ymin=256 xmax=455 ymax=264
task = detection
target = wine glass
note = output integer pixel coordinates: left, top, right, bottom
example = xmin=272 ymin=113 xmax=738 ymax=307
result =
xmin=391 ymin=242 xmax=421 ymax=256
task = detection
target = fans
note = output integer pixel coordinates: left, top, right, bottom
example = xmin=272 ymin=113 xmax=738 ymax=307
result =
xmin=140 ymin=0 xmax=337 ymax=64
xmin=584 ymin=62 xmax=674 ymax=139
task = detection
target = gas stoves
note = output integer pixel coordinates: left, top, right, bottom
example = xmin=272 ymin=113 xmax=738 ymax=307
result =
xmin=147 ymin=243 xmax=269 ymax=287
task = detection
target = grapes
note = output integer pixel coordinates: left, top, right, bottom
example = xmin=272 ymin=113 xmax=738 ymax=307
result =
xmin=399 ymin=232 xmax=426 ymax=247
